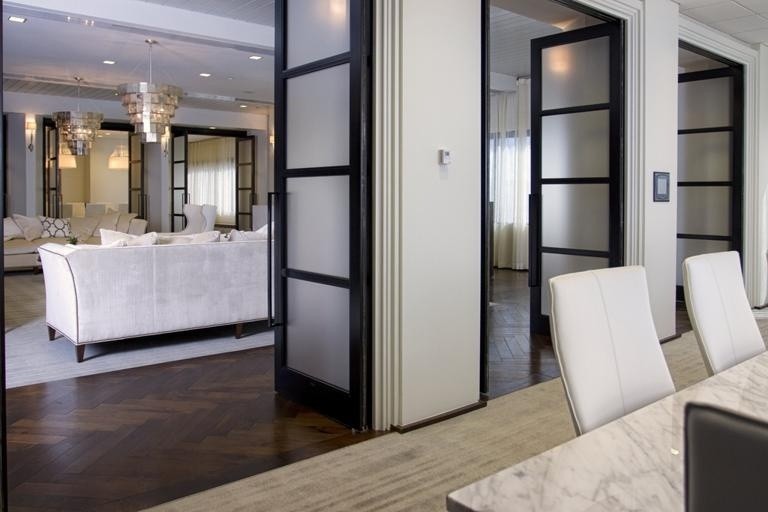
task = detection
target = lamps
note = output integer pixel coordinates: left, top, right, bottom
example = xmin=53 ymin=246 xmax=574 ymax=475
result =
xmin=27 ymin=122 xmax=38 ymax=154
xmin=109 ymin=145 xmax=130 ymax=169
xmin=46 ymin=141 xmax=78 ymax=170
xmin=53 ymin=77 xmax=105 ymax=158
xmin=118 ymin=40 xmax=182 ymax=146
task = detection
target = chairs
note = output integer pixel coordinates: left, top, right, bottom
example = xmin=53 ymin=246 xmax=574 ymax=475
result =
xmin=680 ymin=251 xmax=768 ymax=372
xmin=548 ymin=265 xmax=678 ymax=437
xmin=683 ymin=404 xmax=768 ymax=512
xmin=116 ymin=201 xmax=132 ymax=215
xmin=88 ymin=202 xmax=107 ymax=216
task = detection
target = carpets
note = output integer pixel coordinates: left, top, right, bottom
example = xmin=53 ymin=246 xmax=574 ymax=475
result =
xmin=0 ymin=271 xmax=275 ymax=391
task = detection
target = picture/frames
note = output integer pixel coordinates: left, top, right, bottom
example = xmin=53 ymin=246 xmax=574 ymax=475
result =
xmin=652 ymin=171 xmax=672 ymax=202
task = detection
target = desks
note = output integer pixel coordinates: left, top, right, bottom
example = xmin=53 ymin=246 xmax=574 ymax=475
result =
xmin=445 ymin=349 xmax=768 ymax=512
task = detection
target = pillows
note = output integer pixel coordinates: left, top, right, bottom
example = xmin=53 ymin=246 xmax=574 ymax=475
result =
xmin=117 ymin=212 xmax=139 ymax=235
xmin=38 ymin=214 xmax=71 ymax=239
xmin=0 ymin=217 xmax=25 ymax=244
xmin=227 ymin=221 xmax=275 ymax=241
xmin=98 ymin=226 xmax=158 ymax=248
xmin=72 ymin=216 xmax=93 ymax=239
xmin=173 ymin=231 xmax=223 ymax=245
xmin=13 ymin=213 xmax=45 ymax=242
xmin=93 ymin=212 xmax=121 ymax=238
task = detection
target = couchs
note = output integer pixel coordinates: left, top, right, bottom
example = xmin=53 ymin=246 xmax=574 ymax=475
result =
xmin=37 ymin=243 xmax=273 ymax=363
xmin=2 ymin=216 xmax=148 ymax=275
xmin=149 ymin=203 xmax=216 ymax=246
